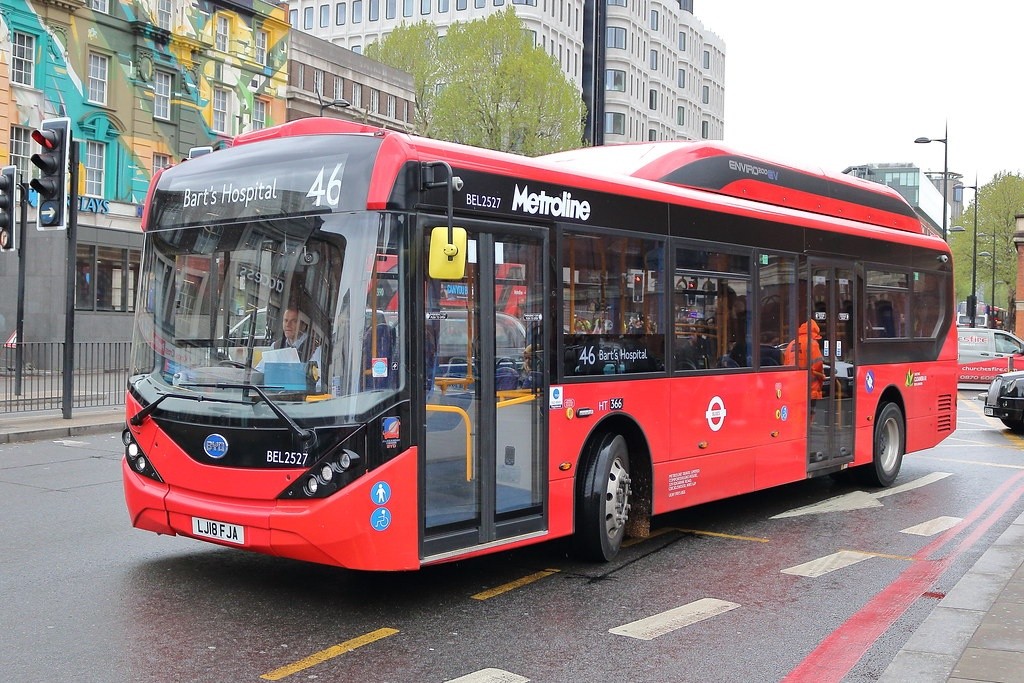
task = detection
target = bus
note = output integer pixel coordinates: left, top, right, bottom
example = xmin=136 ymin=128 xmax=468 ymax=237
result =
xmin=118 ymin=117 xmax=960 ymax=575
xmin=956 ymin=300 xmax=1007 ymax=331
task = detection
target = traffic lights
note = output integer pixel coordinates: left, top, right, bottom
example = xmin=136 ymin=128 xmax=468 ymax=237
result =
xmin=632 ymin=273 xmax=646 ymax=304
xmin=0 ymin=165 xmax=15 ymax=253
xmin=30 ymin=116 xmax=71 ymax=232
xmin=687 ymin=280 xmax=698 ymax=307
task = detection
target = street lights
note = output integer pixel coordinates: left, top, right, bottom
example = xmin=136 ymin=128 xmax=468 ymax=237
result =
xmin=952 ymin=170 xmax=992 ymax=328
xmin=314 ymin=86 xmax=352 ymax=117
xmin=977 ymin=222 xmax=1006 ymax=329
xmin=913 ymin=119 xmax=966 ymax=244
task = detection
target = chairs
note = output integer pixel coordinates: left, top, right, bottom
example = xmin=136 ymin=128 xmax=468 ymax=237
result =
xmin=360 ymin=322 xmax=542 ymax=392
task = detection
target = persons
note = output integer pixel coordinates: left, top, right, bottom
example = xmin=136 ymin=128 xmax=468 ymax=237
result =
xmin=254 ymin=306 xmax=322 ymax=391
xmin=782 ymin=318 xmax=826 ymax=435
xmin=684 ymin=316 xmax=737 ymax=369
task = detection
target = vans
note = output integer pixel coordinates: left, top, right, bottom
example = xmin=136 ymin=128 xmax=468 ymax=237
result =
xmin=956 ymin=327 xmax=1024 ymax=391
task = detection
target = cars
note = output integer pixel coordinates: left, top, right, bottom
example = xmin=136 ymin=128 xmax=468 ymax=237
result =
xmin=978 ymin=371 xmax=1024 ymax=434
xmin=209 ymin=303 xmax=529 ymax=370
